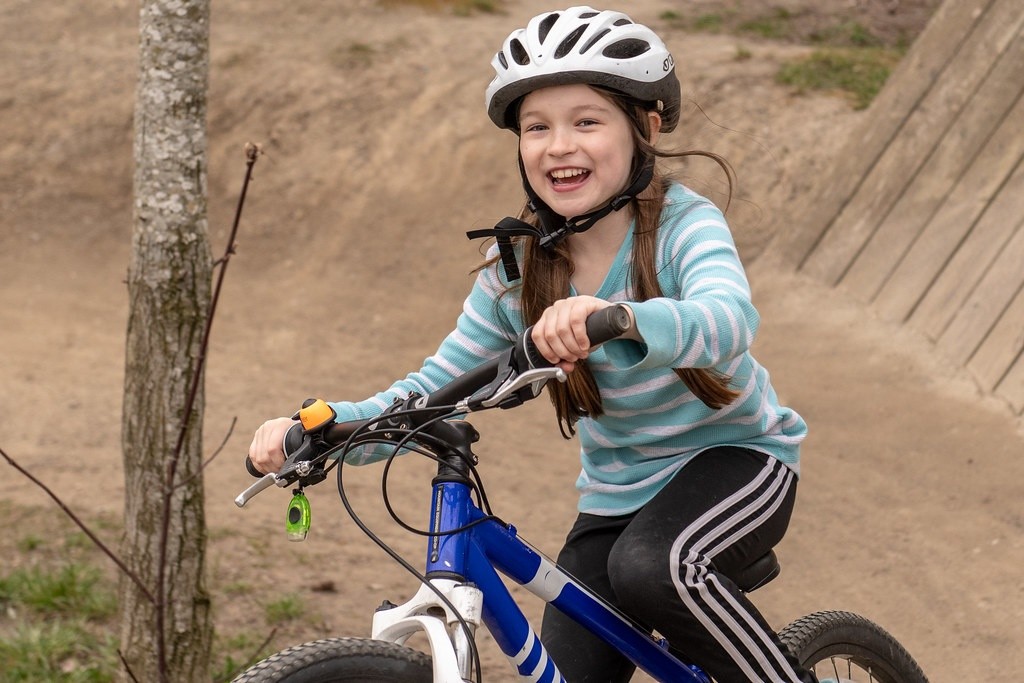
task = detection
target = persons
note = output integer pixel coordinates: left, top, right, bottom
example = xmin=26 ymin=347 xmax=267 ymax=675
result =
xmin=247 ymin=2 xmax=818 ymax=683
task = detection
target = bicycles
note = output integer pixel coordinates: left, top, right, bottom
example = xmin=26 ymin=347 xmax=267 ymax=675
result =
xmin=224 ymin=305 xmax=928 ymax=683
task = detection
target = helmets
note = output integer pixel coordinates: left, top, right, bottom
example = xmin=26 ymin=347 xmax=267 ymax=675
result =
xmin=485 ymin=6 xmax=681 ymax=137
